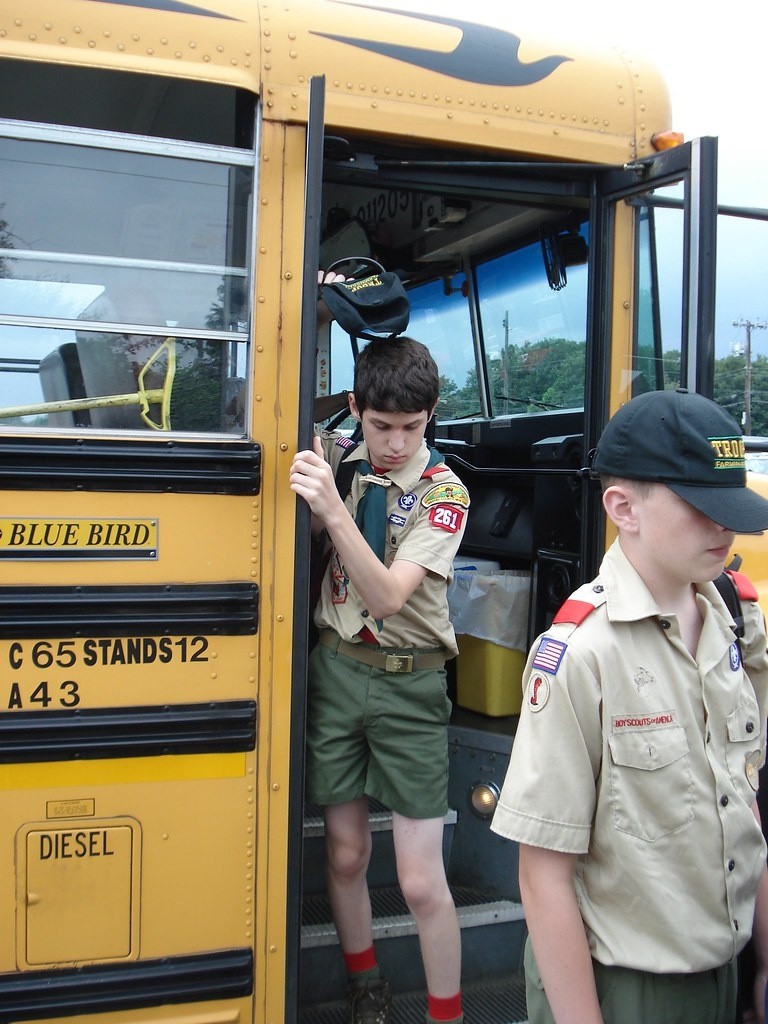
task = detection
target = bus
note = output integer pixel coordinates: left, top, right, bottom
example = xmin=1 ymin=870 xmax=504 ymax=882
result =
xmin=2 ymin=0 xmax=768 ymax=1022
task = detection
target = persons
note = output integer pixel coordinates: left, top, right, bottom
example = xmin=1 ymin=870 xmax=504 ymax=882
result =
xmin=289 ymin=270 xmax=471 ymax=1023
xmin=490 ymin=387 xmax=768 ymax=1024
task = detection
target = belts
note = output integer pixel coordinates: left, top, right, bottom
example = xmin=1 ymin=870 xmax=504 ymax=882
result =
xmin=319 ymin=629 xmax=446 ymax=673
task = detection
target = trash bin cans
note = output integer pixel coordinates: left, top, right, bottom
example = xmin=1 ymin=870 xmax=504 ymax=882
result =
xmin=453 ymin=568 xmax=533 ymax=718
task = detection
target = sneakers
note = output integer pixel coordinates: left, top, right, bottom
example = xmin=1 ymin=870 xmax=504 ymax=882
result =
xmin=346 ymin=976 xmax=393 ymax=1024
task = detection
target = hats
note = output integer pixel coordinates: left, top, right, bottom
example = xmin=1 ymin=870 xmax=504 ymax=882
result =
xmin=594 ymin=387 xmax=768 ymax=534
xmin=321 ymin=268 xmax=410 ymax=342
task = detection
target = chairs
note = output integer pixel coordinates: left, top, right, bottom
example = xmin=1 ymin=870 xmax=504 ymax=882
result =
xmin=74 ymin=288 xmax=244 ymax=431
xmin=38 ymin=342 xmax=89 ymax=429
